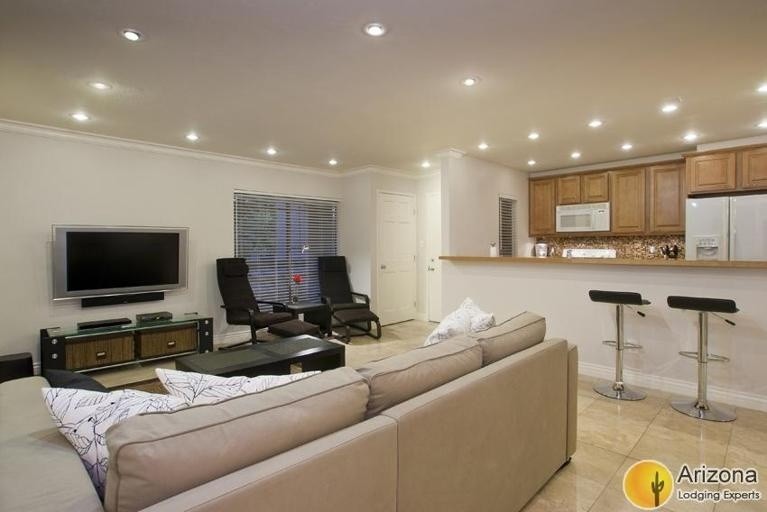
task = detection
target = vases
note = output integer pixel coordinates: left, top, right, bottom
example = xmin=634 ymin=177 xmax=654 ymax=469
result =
xmin=293 ymin=287 xmax=299 ymax=304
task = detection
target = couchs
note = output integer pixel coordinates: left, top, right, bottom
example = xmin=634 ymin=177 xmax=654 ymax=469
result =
xmin=0 ymin=309 xmax=578 ymax=512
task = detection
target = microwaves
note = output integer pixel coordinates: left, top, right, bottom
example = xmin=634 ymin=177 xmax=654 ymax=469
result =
xmin=555 ymin=201 xmax=610 ymax=233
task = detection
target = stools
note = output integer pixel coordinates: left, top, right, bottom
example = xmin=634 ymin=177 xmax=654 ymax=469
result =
xmin=588 ymin=289 xmax=651 ymax=401
xmin=269 ymin=318 xmax=318 ymax=337
xmin=667 ymin=294 xmax=739 ymax=423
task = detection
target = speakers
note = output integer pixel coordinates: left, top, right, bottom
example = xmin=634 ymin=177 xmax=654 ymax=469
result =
xmin=41 ymin=327 xmax=66 ymax=377
xmin=195 ymin=317 xmax=214 ymax=353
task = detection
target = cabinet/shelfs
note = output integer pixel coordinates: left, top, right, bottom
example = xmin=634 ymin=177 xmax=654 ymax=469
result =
xmin=686 ymin=153 xmax=738 ymax=192
xmin=557 ymin=175 xmax=580 ymax=205
xmin=62 ymin=330 xmax=137 ymax=368
xmin=649 ymin=166 xmax=685 ymax=233
xmin=581 ymin=173 xmax=608 ymax=202
xmin=610 ymin=169 xmax=647 ymax=233
xmin=138 ymin=321 xmax=199 ymax=363
xmin=739 ymin=146 xmax=767 ymax=190
xmin=529 ymin=179 xmax=556 ymax=237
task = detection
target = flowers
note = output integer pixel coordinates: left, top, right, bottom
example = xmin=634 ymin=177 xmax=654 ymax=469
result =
xmin=293 ymin=274 xmax=302 ymax=286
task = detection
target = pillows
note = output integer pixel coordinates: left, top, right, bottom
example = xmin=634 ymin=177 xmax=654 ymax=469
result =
xmin=424 ymin=298 xmax=496 ymax=350
xmin=41 ymin=386 xmax=195 ymax=485
xmin=154 ymin=366 xmax=322 ymax=408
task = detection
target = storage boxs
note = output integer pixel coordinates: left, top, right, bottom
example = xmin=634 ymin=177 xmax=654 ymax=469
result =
xmin=174 ymin=335 xmax=346 ymax=379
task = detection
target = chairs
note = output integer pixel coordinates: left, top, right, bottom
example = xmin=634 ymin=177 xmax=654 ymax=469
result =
xmin=317 ymin=254 xmax=383 ymax=343
xmin=215 ymin=257 xmax=293 ymax=351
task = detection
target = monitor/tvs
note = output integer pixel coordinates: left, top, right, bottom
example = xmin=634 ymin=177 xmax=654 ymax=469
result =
xmin=51 ymin=224 xmax=188 ymax=301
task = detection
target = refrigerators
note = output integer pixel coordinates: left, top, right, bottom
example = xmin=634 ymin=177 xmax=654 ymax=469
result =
xmin=684 ymin=194 xmax=766 ymax=262
